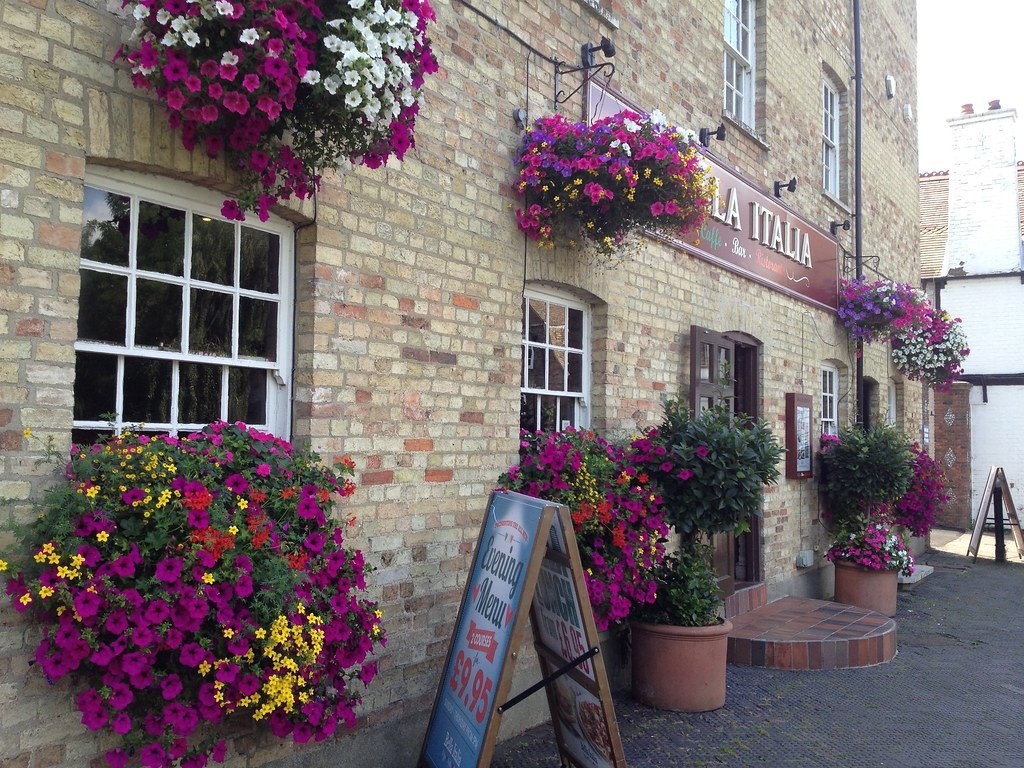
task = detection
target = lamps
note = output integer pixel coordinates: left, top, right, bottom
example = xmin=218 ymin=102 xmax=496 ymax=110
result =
xmin=773 ymin=177 xmax=797 ymax=198
xmin=831 ymin=219 xmax=851 ymax=235
xmin=699 ymin=123 xmax=727 ymax=148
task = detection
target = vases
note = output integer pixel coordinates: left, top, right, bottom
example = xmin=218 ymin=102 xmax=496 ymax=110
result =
xmin=620 ymin=607 xmax=732 ymax=716
xmin=829 ymin=556 xmax=902 ymax=619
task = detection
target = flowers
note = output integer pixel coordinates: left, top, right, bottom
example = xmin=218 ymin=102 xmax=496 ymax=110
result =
xmin=512 ymin=109 xmax=732 ymax=274
xmin=890 ymin=304 xmax=972 ymax=394
xmin=889 ymin=439 xmax=953 ymax=539
xmin=107 ymin=0 xmax=443 ymax=230
xmin=835 ymin=272 xmax=931 ymax=361
xmin=0 ymin=410 xmax=389 ymax=768
xmin=493 ymin=422 xmax=674 ymax=636
xmin=815 ymin=402 xmax=918 ymax=578
xmin=620 ymin=360 xmax=783 ymax=629
